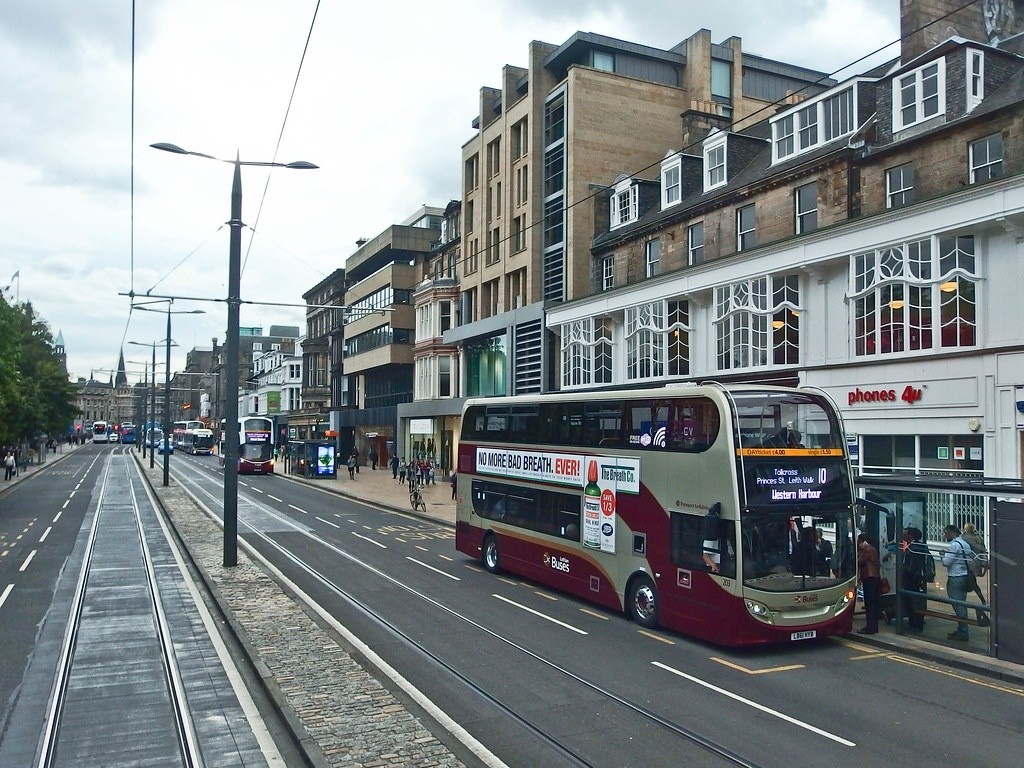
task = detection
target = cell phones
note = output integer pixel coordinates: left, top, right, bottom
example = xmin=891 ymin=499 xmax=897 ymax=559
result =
xmin=859 ymin=541 xmax=868 ymax=546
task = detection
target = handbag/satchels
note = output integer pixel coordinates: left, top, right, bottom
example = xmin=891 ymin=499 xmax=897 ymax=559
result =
xmin=416 ymin=469 xmax=422 ymax=475
xmin=967 ymin=571 xmax=978 ymax=592
xmin=400 ymin=467 xmax=406 ymax=473
xmin=881 ymin=577 xmax=891 ymax=594
xmin=429 ymin=470 xmax=434 ymax=478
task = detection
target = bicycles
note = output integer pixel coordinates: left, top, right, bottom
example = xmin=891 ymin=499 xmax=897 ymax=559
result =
xmin=409 ymin=488 xmax=426 ymax=512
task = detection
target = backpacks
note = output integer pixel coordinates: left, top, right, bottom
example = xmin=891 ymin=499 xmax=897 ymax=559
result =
xmin=925 ymin=552 xmax=936 ymax=582
xmin=979 ymin=551 xmax=989 ymax=578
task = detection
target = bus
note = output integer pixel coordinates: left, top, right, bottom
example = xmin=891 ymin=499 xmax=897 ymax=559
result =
xmin=121 ymin=422 xmax=136 ymax=444
xmin=454 ymin=378 xmax=896 ymax=648
xmin=93 ymin=421 xmax=108 ymax=444
xmin=173 ymin=421 xmax=217 ymax=456
xmin=146 ymin=421 xmax=164 ymax=448
xmin=218 ymin=416 xmax=275 ymax=476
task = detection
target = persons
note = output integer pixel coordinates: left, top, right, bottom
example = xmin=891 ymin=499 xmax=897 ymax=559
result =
xmin=703 ymin=536 xmax=735 ymax=573
xmin=832 ymin=531 xmax=854 ymax=579
xmin=569 ymin=425 xmax=590 ymax=444
xmin=856 ymin=505 xmax=865 ymax=529
xmin=399 ymin=457 xmax=406 ymax=486
xmin=567 ymin=524 xmax=579 ymax=539
xmin=857 ymin=533 xmax=881 ymax=633
xmin=516 ymin=515 xmax=527 ymax=527
xmin=814 ymin=528 xmax=833 ymax=570
xmin=527 ymin=413 xmax=549 ymax=444
xmin=939 ymin=525 xmax=971 ymax=642
xmin=337 ymin=449 xmax=340 ymax=469
xmin=406 ymin=452 xmax=436 ymax=493
xmin=432 ymin=438 xmax=436 ymax=458
xmin=390 ymin=453 xmax=399 ymax=479
xmin=0 ymin=445 xmax=21 ymax=482
xmin=51 ymin=439 xmax=59 ymax=453
xmin=550 ymin=405 xmax=563 ymax=442
xmin=848 ymin=512 xmax=861 ymax=544
xmin=788 ymin=431 xmax=805 ymax=449
xmin=370 ymin=447 xmax=378 ymax=470
xmin=752 ymin=523 xmax=792 ymax=578
xmin=962 ymin=523 xmax=986 ymax=605
xmin=418 ymin=438 xmax=432 ymax=459
xmin=882 ymin=600 xmax=908 ymax=624
xmin=901 ymin=528 xmax=929 ymax=632
xmin=21 ymin=446 xmax=29 ymax=472
xmin=279 ymin=444 xmax=286 ymax=462
xmin=348 ymin=454 xmax=356 ymax=480
xmin=788 ymin=526 xmax=829 ymax=577
xmin=274 ymin=445 xmax=279 ymax=462
xmin=544 ymin=521 xmax=557 ymax=536
xmin=352 ymin=445 xmax=360 ymax=472
xmin=450 ymin=469 xmax=457 ymax=500
xmin=412 ymin=441 xmax=420 ymax=461
xmin=68 ymin=433 xmax=86 ymax=446
xmin=490 ymin=498 xmax=505 ymax=519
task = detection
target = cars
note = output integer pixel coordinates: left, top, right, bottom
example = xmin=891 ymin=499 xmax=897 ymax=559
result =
xmin=109 ymin=433 xmax=119 ymax=443
xmin=158 ymin=439 xmax=174 ymax=454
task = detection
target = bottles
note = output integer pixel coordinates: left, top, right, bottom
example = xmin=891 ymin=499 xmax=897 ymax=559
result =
xmin=583 ymin=459 xmax=602 ymax=549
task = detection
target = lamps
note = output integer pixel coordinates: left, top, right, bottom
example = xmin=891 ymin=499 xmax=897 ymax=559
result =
xmin=941 ymin=282 xmax=959 ymax=293
xmin=889 ymin=299 xmax=904 ymax=309
xmin=773 ymin=321 xmax=783 ymax=329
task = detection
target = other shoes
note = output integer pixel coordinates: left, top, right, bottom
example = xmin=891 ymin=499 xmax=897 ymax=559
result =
xmin=947 ymin=634 xmax=969 ymax=641
xmin=947 ymin=633 xmax=954 ymax=636
xmin=858 ymin=628 xmax=875 ymax=633
xmin=873 ymin=629 xmax=878 ymax=633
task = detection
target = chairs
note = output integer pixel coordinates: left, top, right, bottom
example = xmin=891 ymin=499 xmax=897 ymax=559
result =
xmin=494 ymin=418 xmax=602 ymax=447
xmin=787 ymin=430 xmax=804 ymax=448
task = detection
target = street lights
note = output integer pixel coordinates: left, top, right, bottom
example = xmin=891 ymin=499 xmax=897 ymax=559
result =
xmin=131 ymin=304 xmax=206 ymax=488
xmin=149 ymin=140 xmax=320 ymax=566
xmin=126 ymin=360 xmax=165 ymax=459
xmin=128 ymin=341 xmax=181 ymax=469
